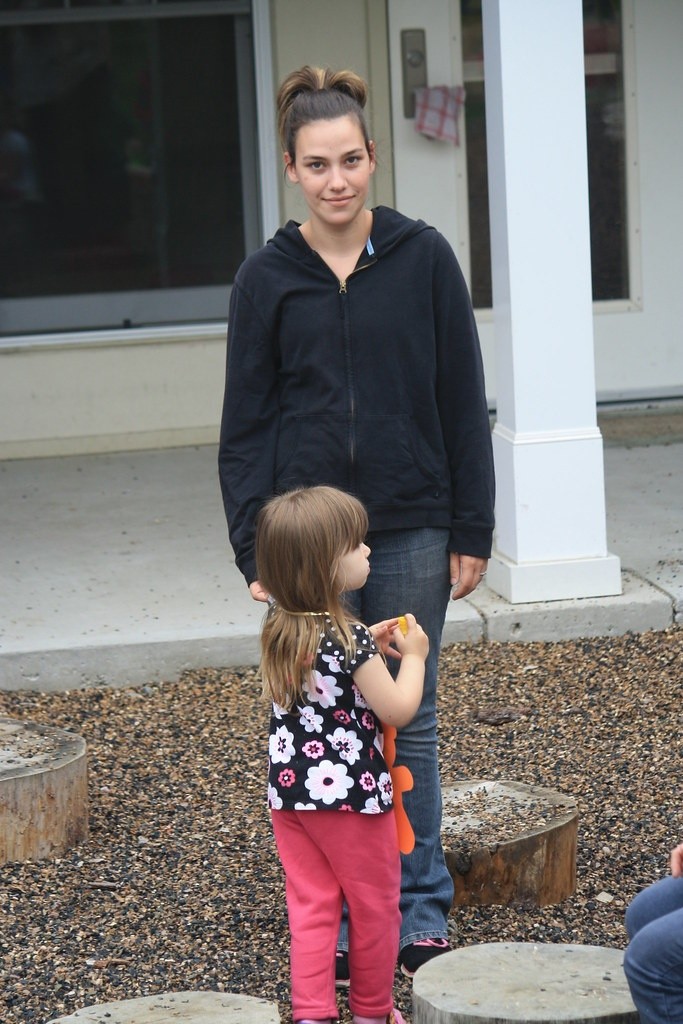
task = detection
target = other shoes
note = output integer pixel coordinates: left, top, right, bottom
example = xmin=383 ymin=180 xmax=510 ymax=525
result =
xmin=335 ymin=951 xmax=350 ymax=988
xmin=401 ymin=938 xmax=452 ymax=978
xmin=388 ymin=1009 xmax=406 ymax=1024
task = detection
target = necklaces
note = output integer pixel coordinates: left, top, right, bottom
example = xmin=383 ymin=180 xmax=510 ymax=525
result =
xmin=279 ymin=605 xmax=331 ymax=616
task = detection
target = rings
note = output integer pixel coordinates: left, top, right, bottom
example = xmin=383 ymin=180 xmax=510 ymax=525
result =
xmin=480 ymin=570 xmax=488 ymax=577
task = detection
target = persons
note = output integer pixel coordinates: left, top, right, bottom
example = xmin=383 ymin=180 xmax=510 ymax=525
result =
xmin=219 ymin=64 xmax=496 ymax=979
xmin=623 ymin=842 xmax=683 ymax=1023
xmin=257 ymin=485 xmax=429 ymax=1024
